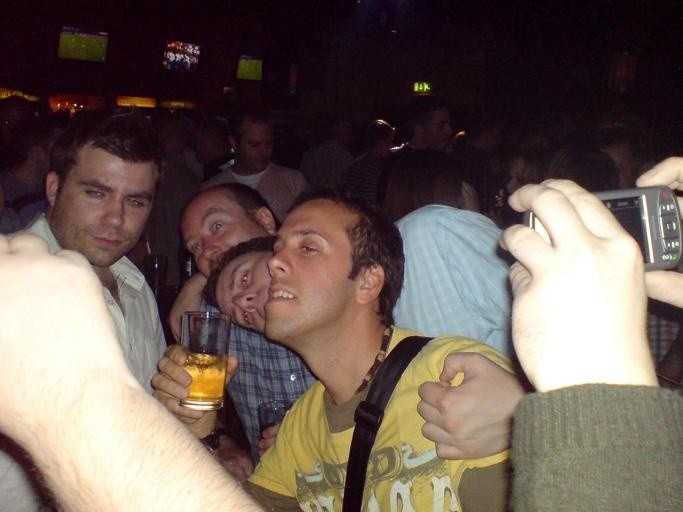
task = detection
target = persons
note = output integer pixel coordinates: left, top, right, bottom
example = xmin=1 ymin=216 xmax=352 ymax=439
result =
xmin=0 ymin=0 xmax=681 ymax=512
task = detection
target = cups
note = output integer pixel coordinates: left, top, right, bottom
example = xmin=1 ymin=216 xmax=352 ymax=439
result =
xmin=177 ymin=311 xmax=232 ymax=412
xmin=258 ymin=399 xmax=292 ymax=440
xmin=144 ymin=255 xmax=167 ymax=304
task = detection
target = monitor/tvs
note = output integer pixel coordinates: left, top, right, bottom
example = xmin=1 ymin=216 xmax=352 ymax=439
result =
xmin=162 ymin=38 xmax=201 ymax=73
xmin=56 ymin=25 xmax=109 ymax=64
xmin=232 ymin=55 xmax=263 ymax=82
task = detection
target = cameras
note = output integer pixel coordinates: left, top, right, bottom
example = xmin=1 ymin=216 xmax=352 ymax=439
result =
xmin=525 ymin=182 xmax=683 ymax=270
